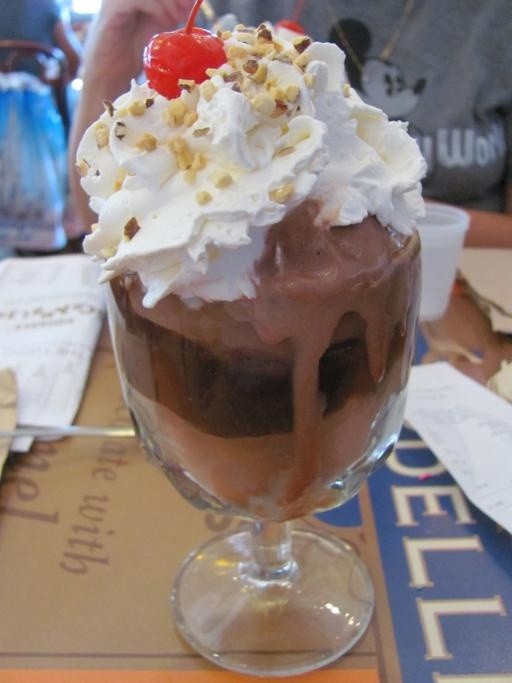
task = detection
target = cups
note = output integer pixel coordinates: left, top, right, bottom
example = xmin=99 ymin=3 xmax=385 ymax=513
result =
xmin=417 ymin=197 xmax=471 ymax=324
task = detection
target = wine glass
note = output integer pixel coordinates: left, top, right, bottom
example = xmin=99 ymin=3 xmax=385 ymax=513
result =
xmin=100 ymin=193 xmax=423 ymax=676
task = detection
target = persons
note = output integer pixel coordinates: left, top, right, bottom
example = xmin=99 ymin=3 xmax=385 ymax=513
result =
xmin=0 ymin=0 xmax=81 ymax=84
xmin=67 ymin=0 xmax=512 ymax=248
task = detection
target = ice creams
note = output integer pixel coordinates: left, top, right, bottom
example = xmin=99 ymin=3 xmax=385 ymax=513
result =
xmin=73 ymin=1 xmax=428 ymax=518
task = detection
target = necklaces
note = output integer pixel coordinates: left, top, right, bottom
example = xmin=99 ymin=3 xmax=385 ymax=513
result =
xmin=325 ymin=0 xmax=416 ymax=85
